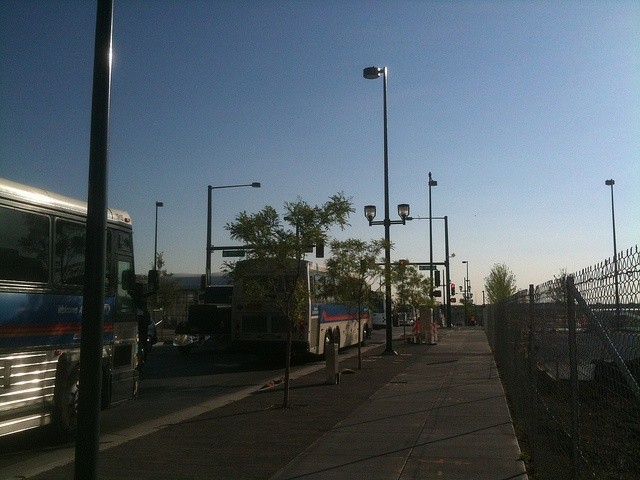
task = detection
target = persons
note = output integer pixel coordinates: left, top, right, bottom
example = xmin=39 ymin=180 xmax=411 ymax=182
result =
xmin=470 ymin=314 xmax=476 ymax=326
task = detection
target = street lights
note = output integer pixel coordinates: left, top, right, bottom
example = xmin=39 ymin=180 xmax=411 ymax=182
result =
xmin=362 ymin=66 xmax=414 ymax=356
xmin=205 ymin=182 xmax=261 ymax=283
xmin=606 ymin=179 xmax=620 ymax=331
xmin=406 ymin=216 xmax=451 ymax=328
xmin=428 ymin=172 xmax=437 ymax=303
xmin=154 ymin=201 xmax=164 ymax=269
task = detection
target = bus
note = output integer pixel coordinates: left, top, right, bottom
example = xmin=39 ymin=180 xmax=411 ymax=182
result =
xmin=229 ymin=258 xmax=373 ymax=361
xmin=371 ymin=290 xmax=394 ymax=328
xmin=0 ymin=177 xmax=141 ymax=443
